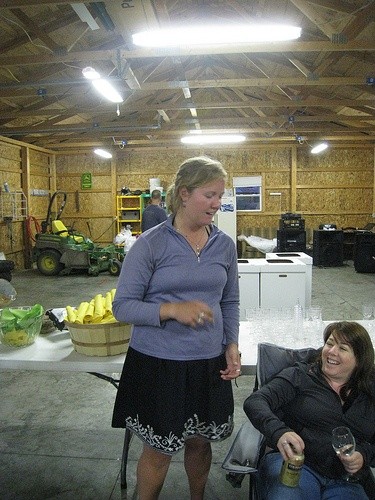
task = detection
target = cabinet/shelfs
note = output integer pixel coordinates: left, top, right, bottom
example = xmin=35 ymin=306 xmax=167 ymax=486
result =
xmin=0 ymin=188 xmax=27 ymax=222
xmin=212 ymin=188 xmax=237 ymax=248
xmin=117 ymin=195 xmax=142 ymax=234
xmin=237 ymin=252 xmax=313 ymax=322
xmin=141 ymin=194 xmax=166 ymax=211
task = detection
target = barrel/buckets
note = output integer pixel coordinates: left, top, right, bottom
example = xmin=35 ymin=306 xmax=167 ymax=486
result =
xmin=63 ymin=313 xmax=134 ymax=358
xmin=149 ymin=179 xmax=161 ymax=194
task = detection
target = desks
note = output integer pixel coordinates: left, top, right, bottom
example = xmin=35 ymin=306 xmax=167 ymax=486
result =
xmin=0 ymin=320 xmax=374 ymax=489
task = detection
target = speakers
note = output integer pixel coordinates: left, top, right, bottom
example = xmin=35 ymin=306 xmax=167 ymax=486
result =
xmin=314 ymin=229 xmax=344 ymax=267
xmin=353 ymin=231 xmax=375 ymax=273
xmin=276 ymin=228 xmax=307 ymax=251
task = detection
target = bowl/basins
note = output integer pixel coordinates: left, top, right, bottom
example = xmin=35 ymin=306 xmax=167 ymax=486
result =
xmin=0 ymin=306 xmax=42 ymax=348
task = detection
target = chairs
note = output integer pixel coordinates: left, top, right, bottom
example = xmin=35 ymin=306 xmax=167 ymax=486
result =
xmin=221 ymin=342 xmax=375 ymax=500
xmin=52 ymin=220 xmax=83 ymax=243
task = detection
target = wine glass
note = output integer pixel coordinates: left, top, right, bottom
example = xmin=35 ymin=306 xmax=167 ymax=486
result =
xmin=331 ymin=426 xmax=361 ymax=482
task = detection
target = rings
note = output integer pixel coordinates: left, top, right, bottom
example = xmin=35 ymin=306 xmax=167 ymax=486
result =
xmin=198 ymin=313 xmax=205 ymax=322
xmin=281 ymin=441 xmax=288 ymax=446
xmin=236 ymin=369 xmax=240 ymax=372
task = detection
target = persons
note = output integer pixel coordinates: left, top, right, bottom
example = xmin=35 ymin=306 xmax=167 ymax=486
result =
xmin=112 ymin=158 xmax=241 ymax=500
xmin=242 ymin=322 xmax=375 ymax=500
xmin=141 ymin=190 xmax=167 ymax=234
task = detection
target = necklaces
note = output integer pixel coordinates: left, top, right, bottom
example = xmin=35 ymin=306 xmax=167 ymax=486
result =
xmin=178 ymin=229 xmax=206 ymax=254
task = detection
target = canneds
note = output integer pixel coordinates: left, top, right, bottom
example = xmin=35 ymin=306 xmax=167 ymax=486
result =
xmin=280 ymin=446 xmax=304 ymax=487
